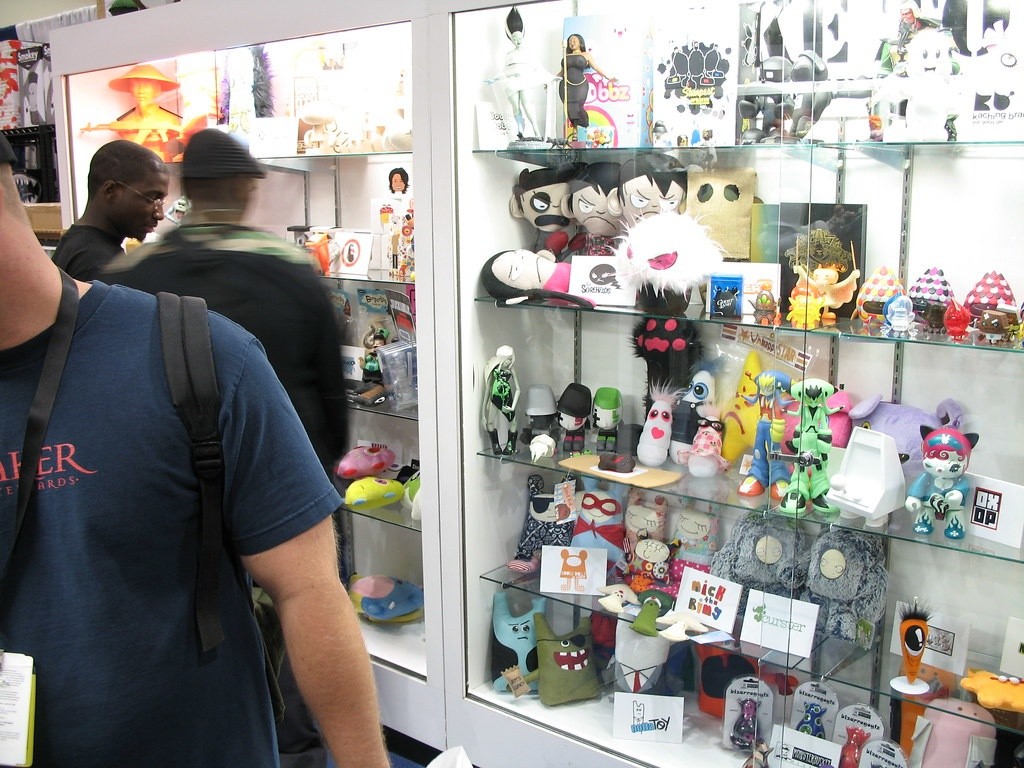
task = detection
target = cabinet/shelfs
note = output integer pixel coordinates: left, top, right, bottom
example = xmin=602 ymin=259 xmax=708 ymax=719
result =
xmin=464 ymin=137 xmax=1023 ymax=766
xmin=57 ymin=151 xmax=428 ymax=685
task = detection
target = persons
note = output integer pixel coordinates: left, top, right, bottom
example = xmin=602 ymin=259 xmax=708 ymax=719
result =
xmin=0 ymin=129 xmax=392 ymax=768
xmin=898 ymin=1 xmax=960 ymax=141
xmin=479 ymin=152 xmax=757 ymax=316
xmin=481 ymin=345 xmax=521 ymax=455
xmin=551 ymin=33 xmax=622 ymax=141
xmin=109 ymin=64 xmax=183 ymax=162
xmin=51 ymin=129 xmax=351 ymax=768
xmin=482 ymin=5 xmax=563 ymax=140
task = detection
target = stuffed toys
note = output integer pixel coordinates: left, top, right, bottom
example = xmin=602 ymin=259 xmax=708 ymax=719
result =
xmin=348 ymin=572 xmax=424 ymax=623
xmin=506 ymin=474 xmax=890 ymax=645
xmin=336 ymin=446 xmax=421 ymax=521
xmin=614 ymin=211 xmax=763 ymax=479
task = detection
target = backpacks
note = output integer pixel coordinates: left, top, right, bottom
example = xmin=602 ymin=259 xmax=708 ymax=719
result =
xmin=156 ymin=290 xmax=288 ymax=729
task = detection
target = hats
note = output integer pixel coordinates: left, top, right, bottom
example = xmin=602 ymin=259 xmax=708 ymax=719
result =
xmin=182 ymin=128 xmax=267 ymax=179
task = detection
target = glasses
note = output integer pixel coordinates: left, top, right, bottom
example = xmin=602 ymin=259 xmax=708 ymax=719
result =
xmin=114 ymin=179 xmax=169 ymax=210
